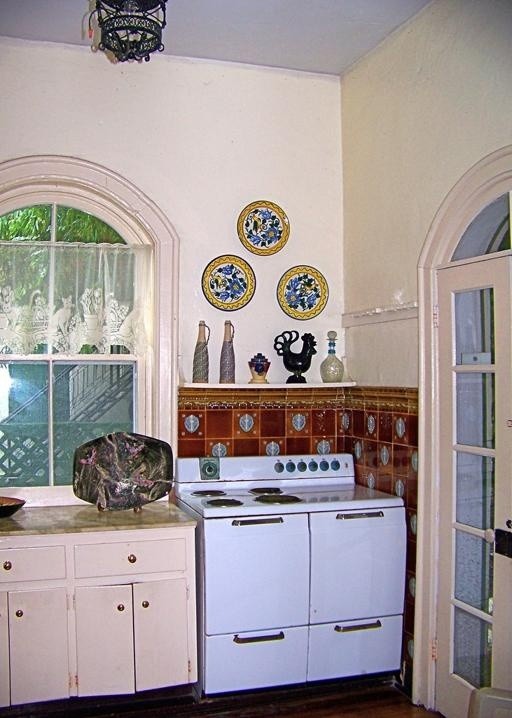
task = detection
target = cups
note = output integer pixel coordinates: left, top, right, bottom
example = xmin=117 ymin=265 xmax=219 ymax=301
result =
xmin=246 ymin=352 xmax=272 ymax=385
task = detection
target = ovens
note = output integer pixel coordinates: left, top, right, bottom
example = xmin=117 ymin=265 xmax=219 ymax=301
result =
xmin=200 ymin=506 xmax=407 ymax=700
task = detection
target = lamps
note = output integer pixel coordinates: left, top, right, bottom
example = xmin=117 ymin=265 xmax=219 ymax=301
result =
xmin=86 ymin=0 xmax=168 ymax=61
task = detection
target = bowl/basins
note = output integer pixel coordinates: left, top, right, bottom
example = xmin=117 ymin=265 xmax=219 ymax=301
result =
xmin=0 ymin=496 xmax=28 ymax=518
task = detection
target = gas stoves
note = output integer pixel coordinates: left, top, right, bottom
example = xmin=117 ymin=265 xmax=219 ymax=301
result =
xmin=174 ymin=476 xmax=408 ymax=519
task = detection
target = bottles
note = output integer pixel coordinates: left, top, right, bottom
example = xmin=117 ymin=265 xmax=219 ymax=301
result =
xmin=218 ymin=320 xmax=236 ymax=384
xmin=192 ymin=320 xmax=210 ymax=383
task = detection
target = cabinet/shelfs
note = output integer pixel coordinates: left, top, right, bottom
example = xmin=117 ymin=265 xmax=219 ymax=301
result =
xmin=0 ymin=526 xmax=197 ymax=712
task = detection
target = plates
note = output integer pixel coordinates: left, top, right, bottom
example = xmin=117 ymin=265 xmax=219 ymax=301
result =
xmin=71 ymin=429 xmax=175 ymax=512
